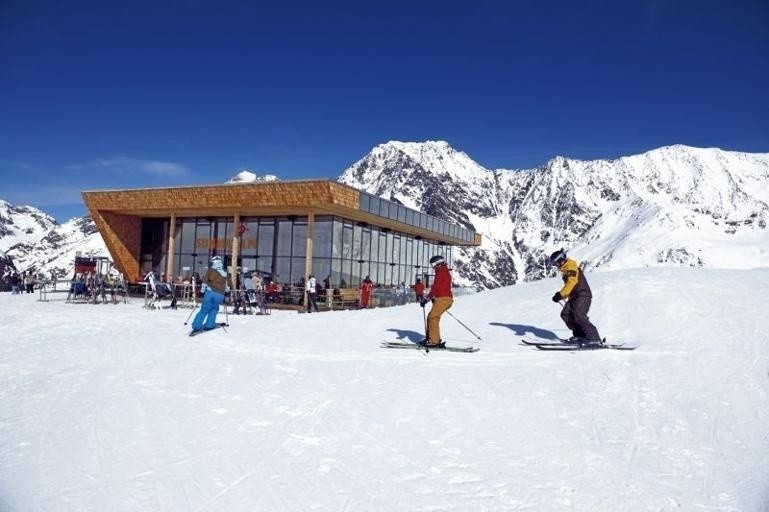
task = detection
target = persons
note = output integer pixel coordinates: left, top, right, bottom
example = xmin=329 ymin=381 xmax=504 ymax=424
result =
xmin=421 ymin=255 xmax=454 ymax=347
xmin=11 ymin=271 xmax=37 ymax=294
xmin=306 ymin=273 xmax=319 ymax=313
xmin=550 ymin=250 xmax=603 ymax=347
xmin=359 ymin=276 xmax=372 ymax=309
xmin=413 ymin=279 xmax=424 ymax=303
xmin=191 ymin=255 xmax=230 ymax=331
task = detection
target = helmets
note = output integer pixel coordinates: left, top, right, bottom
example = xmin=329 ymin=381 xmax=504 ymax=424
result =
xmin=430 ymin=256 xmax=444 ymax=268
xmin=551 ymin=251 xmax=566 ymax=264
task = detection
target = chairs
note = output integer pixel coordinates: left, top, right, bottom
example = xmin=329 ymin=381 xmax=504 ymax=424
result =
xmin=76 ymin=275 xmax=288 ymax=314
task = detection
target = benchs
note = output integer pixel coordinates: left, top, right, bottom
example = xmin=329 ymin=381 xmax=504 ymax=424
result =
xmin=338 ymin=287 xmax=361 ymax=308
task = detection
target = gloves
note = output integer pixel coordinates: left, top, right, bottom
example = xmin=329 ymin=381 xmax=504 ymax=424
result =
xmin=553 ymin=292 xmax=561 ymax=302
xmin=420 ymin=297 xmax=429 ymax=306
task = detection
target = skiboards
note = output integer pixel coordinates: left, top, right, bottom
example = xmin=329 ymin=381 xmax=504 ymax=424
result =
xmin=189 ymin=322 xmax=227 ymax=336
xmin=522 ymin=336 xmax=642 ymax=351
xmin=381 ymin=339 xmax=480 ymax=353
